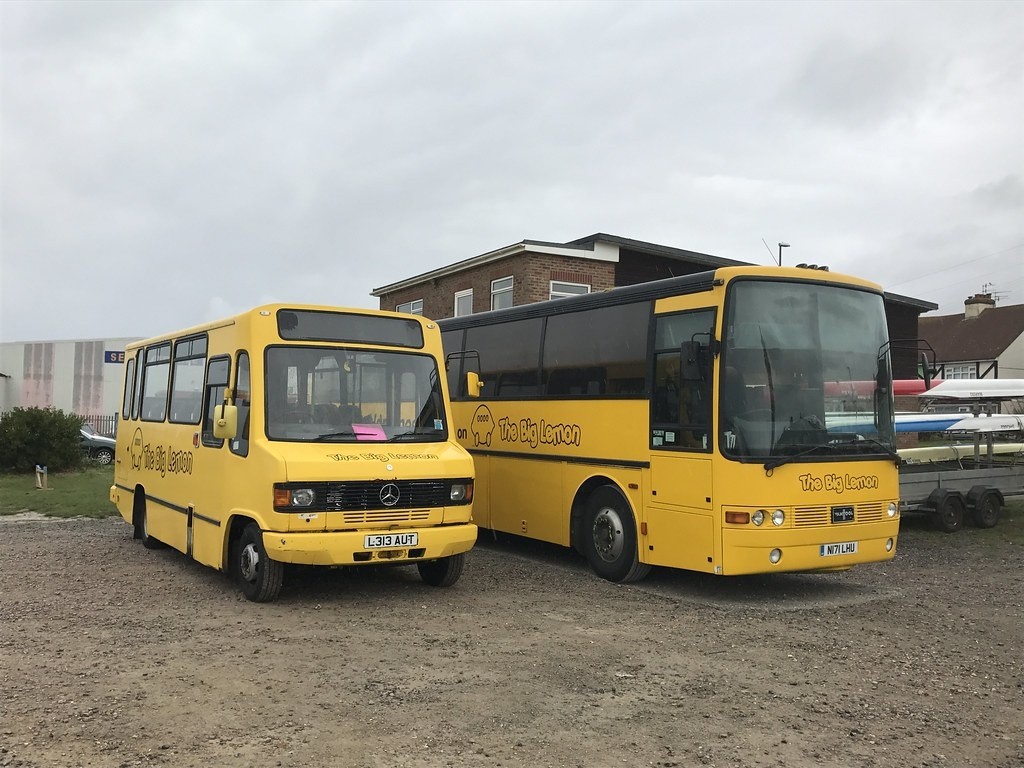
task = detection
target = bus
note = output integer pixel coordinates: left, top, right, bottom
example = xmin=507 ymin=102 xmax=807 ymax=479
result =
xmin=309 ymin=264 xmax=938 ymax=584
xmin=110 ymin=305 xmax=487 ymax=604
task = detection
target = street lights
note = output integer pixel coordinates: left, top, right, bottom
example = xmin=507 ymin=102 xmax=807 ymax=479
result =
xmin=778 ymin=241 xmax=791 ymax=266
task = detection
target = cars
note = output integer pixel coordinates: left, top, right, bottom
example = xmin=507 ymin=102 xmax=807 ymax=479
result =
xmin=78 ymin=429 xmax=116 ymax=466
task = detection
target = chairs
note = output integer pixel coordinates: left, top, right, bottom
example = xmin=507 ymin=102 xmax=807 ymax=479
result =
xmin=131 ymin=402 xmax=365 ymax=428
xmin=461 ymin=365 xmax=608 ymax=397
